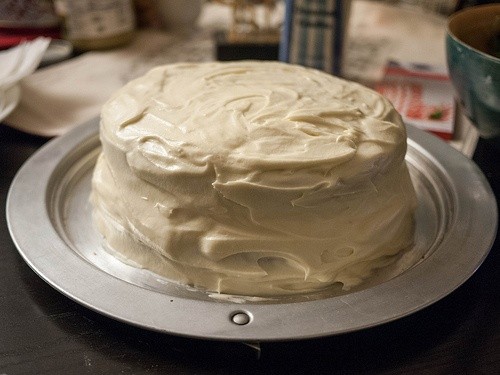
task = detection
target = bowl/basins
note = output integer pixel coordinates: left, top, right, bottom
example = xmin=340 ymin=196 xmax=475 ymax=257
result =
xmin=446 ymin=1 xmax=500 ymax=142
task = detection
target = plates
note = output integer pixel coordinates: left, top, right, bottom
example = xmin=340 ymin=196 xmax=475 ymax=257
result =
xmin=4 ymin=114 xmax=497 ymax=341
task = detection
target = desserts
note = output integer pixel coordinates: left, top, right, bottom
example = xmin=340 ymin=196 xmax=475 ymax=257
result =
xmin=88 ymin=61 xmax=420 ymax=297
